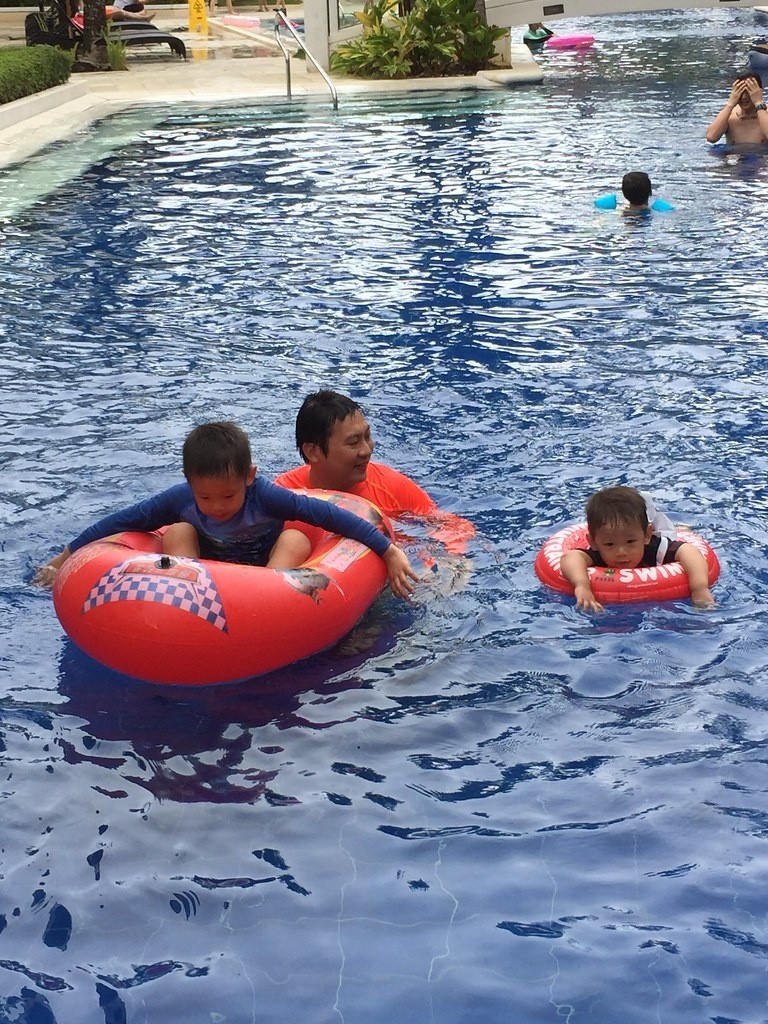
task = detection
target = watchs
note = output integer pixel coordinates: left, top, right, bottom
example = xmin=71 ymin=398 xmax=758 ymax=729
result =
xmin=756 ymin=103 xmax=767 ymax=112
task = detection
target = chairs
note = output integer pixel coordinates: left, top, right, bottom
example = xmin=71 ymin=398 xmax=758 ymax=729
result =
xmin=25 ymin=12 xmax=187 ymax=61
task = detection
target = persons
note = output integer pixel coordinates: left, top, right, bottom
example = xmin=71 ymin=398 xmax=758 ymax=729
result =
xmin=258 ymin=0 xmax=286 ymax=12
xmin=706 ymin=72 xmax=768 ymax=143
xmin=48 ymin=423 xmax=421 ymax=599
xmin=559 ymin=487 xmax=714 ymax=613
xmin=59 ymin=0 xmax=156 ymax=23
xmin=210 ymin=0 xmax=239 ymax=17
xmin=270 ymin=390 xmax=434 ymax=516
xmin=523 ymin=23 xmax=557 ymax=46
xmin=622 ymin=172 xmax=652 ymax=210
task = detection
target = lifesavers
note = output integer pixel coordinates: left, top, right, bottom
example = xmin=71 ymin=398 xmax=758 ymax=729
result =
xmin=51 ymin=486 xmax=394 ymax=685
xmin=534 ymin=519 xmax=721 ymax=604
xmin=546 ymin=35 xmax=595 ymax=47
